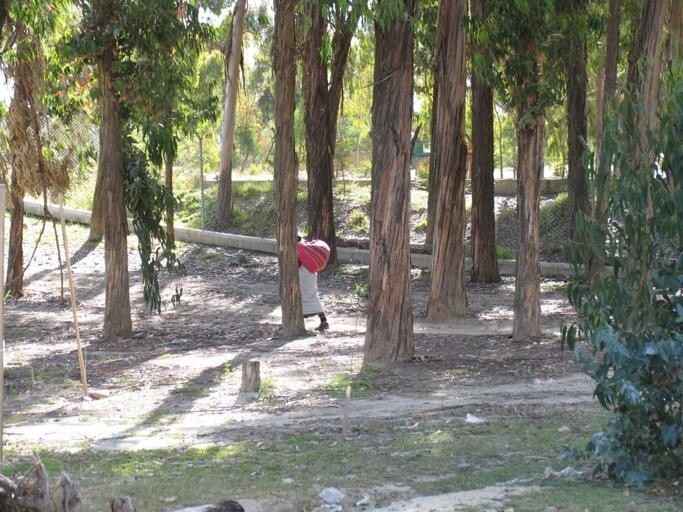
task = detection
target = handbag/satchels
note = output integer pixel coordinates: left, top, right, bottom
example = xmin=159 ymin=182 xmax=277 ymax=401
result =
xmin=298 ymin=239 xmax=330 ymax=273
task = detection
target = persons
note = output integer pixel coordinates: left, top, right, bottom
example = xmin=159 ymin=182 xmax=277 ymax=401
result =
xmin=297 ymin=236 xmax=329 ymax=331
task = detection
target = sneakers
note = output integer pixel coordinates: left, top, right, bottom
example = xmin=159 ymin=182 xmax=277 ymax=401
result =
xmin=316 ymin=321 xmax=329 ymax=330
